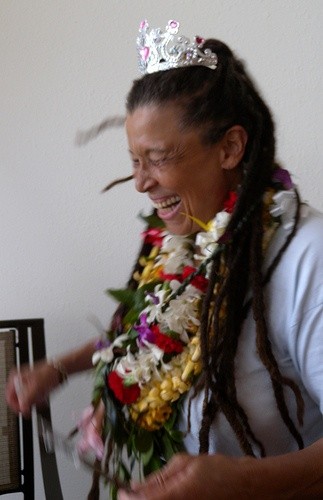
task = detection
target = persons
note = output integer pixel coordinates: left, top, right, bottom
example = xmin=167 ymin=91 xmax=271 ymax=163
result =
xmin=9 ymin=39 xmax=322 ymax=499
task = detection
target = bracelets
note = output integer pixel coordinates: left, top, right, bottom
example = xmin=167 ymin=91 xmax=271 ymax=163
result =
xmin=46 ymin=358 xmax=68 ymax=384
